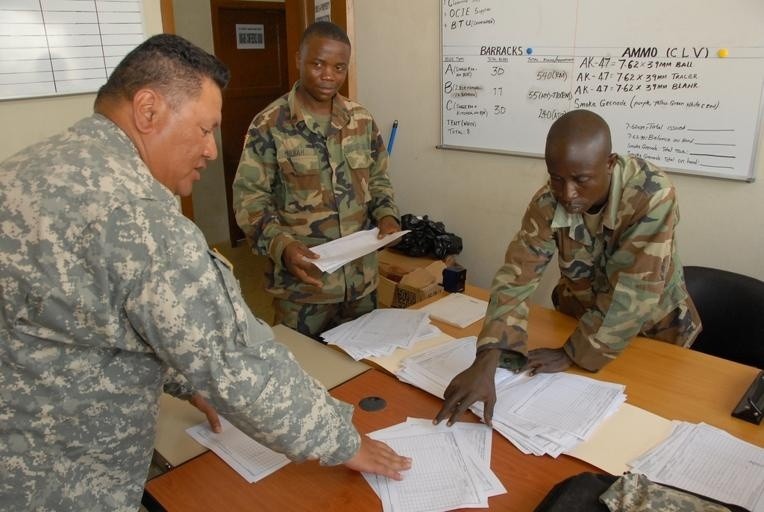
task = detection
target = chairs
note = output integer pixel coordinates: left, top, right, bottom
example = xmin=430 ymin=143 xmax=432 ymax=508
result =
xmin=682 ymin=266 xmax=763 ymax=370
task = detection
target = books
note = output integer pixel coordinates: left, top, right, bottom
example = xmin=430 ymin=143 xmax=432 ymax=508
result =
xmin=418 ymin=292 xmax=490 ymax=329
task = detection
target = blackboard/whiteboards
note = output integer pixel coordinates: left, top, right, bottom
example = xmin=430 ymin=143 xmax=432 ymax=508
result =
xmin=443 ymin=0 xmax=764 ymax=180
xmin=0 ymin=0 xmax=147 ymax=99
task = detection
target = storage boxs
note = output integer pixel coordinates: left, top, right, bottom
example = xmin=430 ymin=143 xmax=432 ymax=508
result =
xmin=377 ymin=248 xmax=455 ymax=308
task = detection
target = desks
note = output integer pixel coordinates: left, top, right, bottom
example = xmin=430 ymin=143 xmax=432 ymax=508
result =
xmin=144 ymin=368 xmax=618 ymax=512
xmin=327 ymin=285 xmax=764 ymax=473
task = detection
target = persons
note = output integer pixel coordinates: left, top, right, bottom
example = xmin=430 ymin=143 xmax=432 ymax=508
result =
xmin=231 ymin=21 xmax=401 ymax=341
xmin=0 ymin=34 xmax=412 ymax=512
xmin=431 ymin=110 xmax=703 ymax=428
